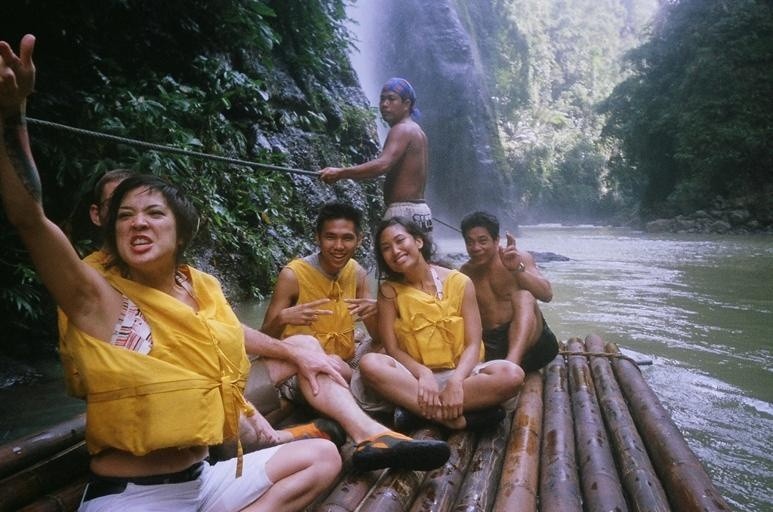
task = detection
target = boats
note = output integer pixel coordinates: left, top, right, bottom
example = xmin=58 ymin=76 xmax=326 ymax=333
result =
xmin=0 ymin=327 xmax=737 ymax=512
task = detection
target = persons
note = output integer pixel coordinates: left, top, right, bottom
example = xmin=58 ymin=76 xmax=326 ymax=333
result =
xmin=319 ymin=76 xmax=433 ymax=262
xmin=455 ymin=211 xmax=559 ymax=372
xmin=0 ymin=34 xmax=343 ymax=512
xmin=58 ymin=169 xmax=452 ymax=471
xmin=356 ymin=215 xmax=524 ymax=434
xmin=254 ymin=202 xmax=387 ymax=405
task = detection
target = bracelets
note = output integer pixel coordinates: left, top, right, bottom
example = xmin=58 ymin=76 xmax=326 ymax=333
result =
xmin=519 ymin=262 xmax=525 ymax=272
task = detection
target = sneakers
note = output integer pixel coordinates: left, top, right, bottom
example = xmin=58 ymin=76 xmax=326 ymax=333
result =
xmin=463 ymin=405 xmax=507 ymax=431
xmin=276 ymin=417 xmax=347 ymax=448
xmin=352 ymin=433 xmax=452 ymax=471
xmin=392 ymin=406 xmax=413 ymax=431
